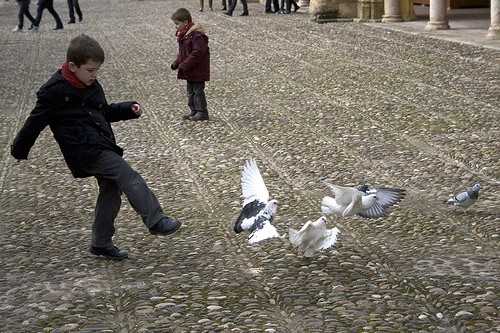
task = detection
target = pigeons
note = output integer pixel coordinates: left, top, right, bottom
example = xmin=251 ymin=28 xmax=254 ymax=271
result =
xmin=355 ymin=185 xmax=407 ymax=219
xmin=233 ymin=157 xmax=285 ymax=245
xmin=288 ymin=215 xmax=341 ymax=258
xmin=320 ymin=181 xmax=381 ymax=218
xmin=441 ymin=183 xmax=484 ymax=216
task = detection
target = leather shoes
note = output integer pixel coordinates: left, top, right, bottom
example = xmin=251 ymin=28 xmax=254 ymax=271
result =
xmin=149 ymin=216 xmax=182 ymax=235
xmin=89 ymin=245 xmax=128 ymax=260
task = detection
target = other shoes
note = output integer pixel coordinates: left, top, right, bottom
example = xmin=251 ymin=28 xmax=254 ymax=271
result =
xmin=221 ymin=7 xmax=227 ymax=10
xmin=275 ymin=9 xmax=285 ymax=14
xmin=67 ymin=20 xmax=75 ymax=24
xmin=200 ymin=8 xmax=203 ymax=11
xmin=29 ymin=27 xmax=40 ymax=31
xmin=240 ymin=10 xmax=249 ymax=16
xmin=27 ymin=24 xmax=34 ymax=30
xmin=182 ymin=110 xmax=197 ymax=118
xmin=209 ymin=7 xmax=212 ymax=11
xmin=12 ymin=26 xmax=22 ymax=32
xmin=294 ymin=6 xmax=300 ymax=12
xmin=79 ymin=17 xmax=83 ymax=21
xmin=189 ymin=111 xmax=209 ymax=120
xmin=53 ymin=26 xmax=63 ymax=30
xmin=265 ymin=10 xmax=275 ymax=13
xmin=223 ymin=9 xmax=233 ymax=16
xmin=285 ymin=10 xmax=291 ymax=14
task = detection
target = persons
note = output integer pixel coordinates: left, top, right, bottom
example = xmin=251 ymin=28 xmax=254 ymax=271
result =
xmin=28 ymin=0 xmax=63 ymax=30
xmin=66 ymin=0 xmax=83 ymax=24
xmin=5 ymin=0 xmax=40 ymax=32
xmin=10 ymin=33 xmax=181 ymax=260
xmin=170 ymin=7 xmax=210 ymax=121
xmin=199 ymin=0 xmax=300 ymax=17
